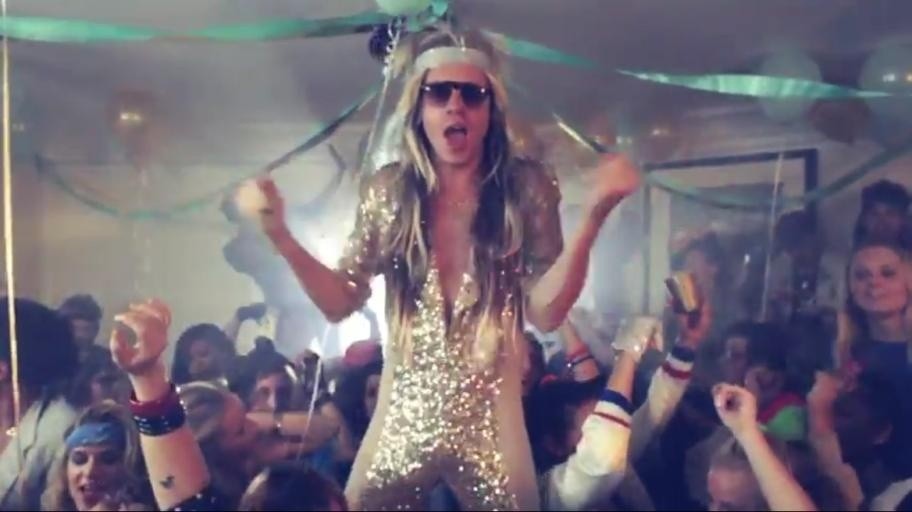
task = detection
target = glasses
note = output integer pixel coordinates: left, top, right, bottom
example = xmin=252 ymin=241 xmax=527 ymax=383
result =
xmin=419 ymin=82 xmax=490 ymax=105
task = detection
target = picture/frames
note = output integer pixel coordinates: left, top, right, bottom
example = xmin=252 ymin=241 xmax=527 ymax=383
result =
xmin=644 ymin=147 xmax=819 ymax=410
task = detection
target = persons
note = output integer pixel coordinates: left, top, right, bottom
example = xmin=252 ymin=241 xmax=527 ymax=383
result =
xmin=251 ymin=24 xmax=645 ymax=511
xmin=0 ymin=182 xmax=912 ymax=512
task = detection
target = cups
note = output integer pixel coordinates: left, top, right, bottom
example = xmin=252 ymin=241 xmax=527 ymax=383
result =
xmin=664 ymin=272 xmax=702 ymax=314
